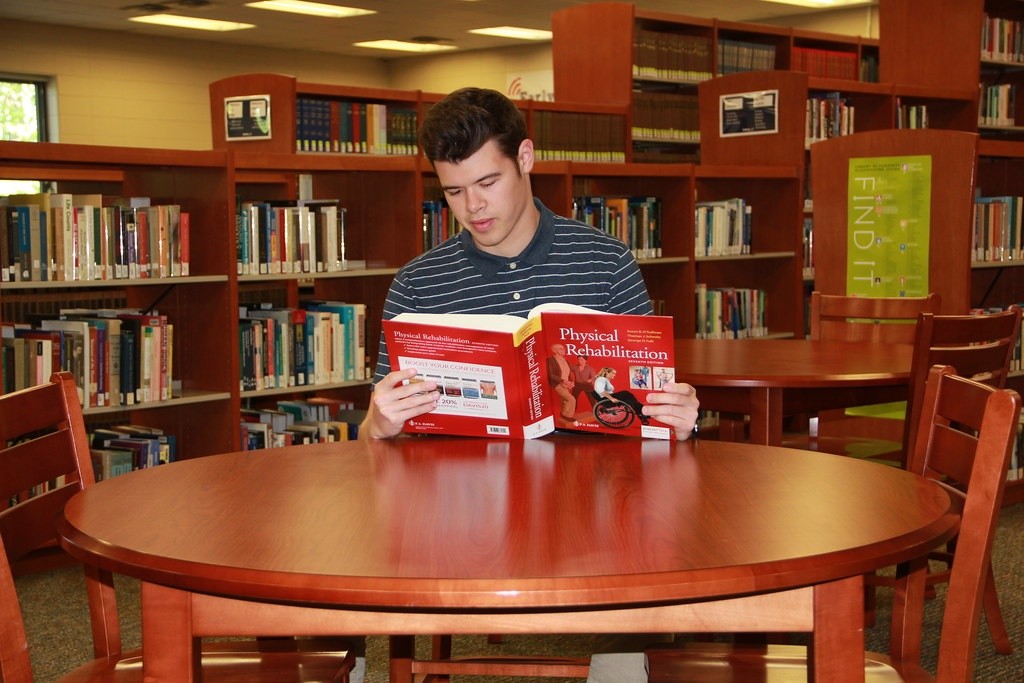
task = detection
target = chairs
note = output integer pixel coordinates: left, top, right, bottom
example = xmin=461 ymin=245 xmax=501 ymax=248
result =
xmin=0 ymin=249 xmax=1020 ymax=683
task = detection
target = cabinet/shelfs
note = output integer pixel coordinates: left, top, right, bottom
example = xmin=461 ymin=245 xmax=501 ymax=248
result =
xmin=0 ymin=0 xmax=1024 ymax=506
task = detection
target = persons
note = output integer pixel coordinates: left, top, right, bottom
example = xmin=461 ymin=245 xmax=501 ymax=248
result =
xmin=569 ymin=354 xmax=599 ymax=414
xmin=589 ymin=367 xmax=653 ymax=426
xmin=546 ymin=344 xmax=579 ymax=422
xmin=635 ymin=368 xmax=648 ymax=388
xmin=358 ymin=86 xmax=699 ymax=441
xmin=658 ymin=368 xmax=668 ymax=388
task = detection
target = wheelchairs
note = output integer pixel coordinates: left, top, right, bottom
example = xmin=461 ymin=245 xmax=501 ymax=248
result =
xmin=591 ymin=390 xmax=649 ymax=429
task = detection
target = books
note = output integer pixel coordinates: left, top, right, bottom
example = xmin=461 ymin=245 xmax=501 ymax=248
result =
xmin=419 ymin=196 xmax=667 ymax=314
xmin=633 ymin=11 xmax=1024 ymax=484
xmin=295 ymin=92 xmax=626 ymax=164
xmin=0 ymin=174 xmax=372 ymax=539
xmin=382 ymin=302 xmax=680 ymax=441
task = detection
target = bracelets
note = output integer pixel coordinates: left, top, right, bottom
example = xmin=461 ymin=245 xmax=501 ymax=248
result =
xmin=688 ymin=423 xmax=699 ymax=440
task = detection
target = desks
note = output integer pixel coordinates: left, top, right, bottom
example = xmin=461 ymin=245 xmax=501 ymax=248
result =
xmin=672 ymin=338 xmax=917 ymax=448
xmin=60 ymin=432 xmax=947 ymax=683
